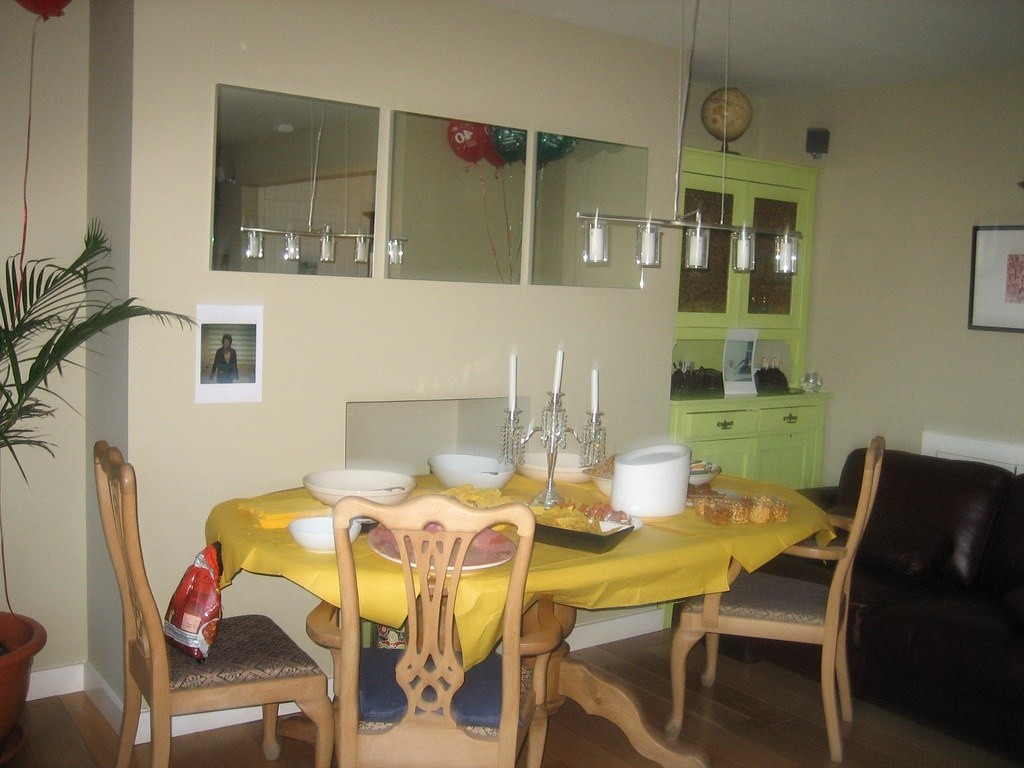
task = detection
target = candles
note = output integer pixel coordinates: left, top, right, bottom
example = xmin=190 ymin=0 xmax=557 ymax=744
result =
xmin=553 ymin=350 xmax=564 ymax=404
xmin=508 ymin=354 xmax=517 ymax=420
xmin=591 ymin=367 xmax=599 ymax=414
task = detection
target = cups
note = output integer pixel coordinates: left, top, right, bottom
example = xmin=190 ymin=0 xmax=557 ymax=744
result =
xmin=801 ymin=371 xmax=823 ymax=394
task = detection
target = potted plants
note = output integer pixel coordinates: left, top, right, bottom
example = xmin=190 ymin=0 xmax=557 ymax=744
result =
xmin=0 ymin=222 xmax=196 ymax=739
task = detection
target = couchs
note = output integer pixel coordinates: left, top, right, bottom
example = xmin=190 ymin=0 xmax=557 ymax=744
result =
xmin=671 ymin=450 xmax=1024 ymax=763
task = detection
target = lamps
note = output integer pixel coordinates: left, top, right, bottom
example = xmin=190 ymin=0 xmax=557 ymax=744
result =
xmin=241 ymin=98 xmax=408 ymax=264
xmin=575 ymin=0 xmax=803 ymax=271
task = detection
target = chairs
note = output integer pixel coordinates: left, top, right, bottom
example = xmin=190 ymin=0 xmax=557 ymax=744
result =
xmin=333 ymin=493 xmax=539 ymax=768
xmin=662 ymin=437 xmax=884 ymax=762
xmin=93 ymin=439 xmax=334 ymax=768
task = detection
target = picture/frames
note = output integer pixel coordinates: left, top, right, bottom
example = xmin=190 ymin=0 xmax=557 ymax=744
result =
xmin=967 ymin=225 xmax=1024 ymax=334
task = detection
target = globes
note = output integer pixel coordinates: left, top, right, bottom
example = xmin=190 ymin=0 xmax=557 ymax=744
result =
xmin=701 ymin=86 xmax=753 ymax=155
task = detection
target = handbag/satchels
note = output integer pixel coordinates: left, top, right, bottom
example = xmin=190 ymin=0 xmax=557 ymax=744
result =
xmin=162 ymin=542 xmax=223 ymax=663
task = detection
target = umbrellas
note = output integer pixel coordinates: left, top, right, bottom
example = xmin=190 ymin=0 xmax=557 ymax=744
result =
xmin=448 ymin=120 xmax=490 ymax=163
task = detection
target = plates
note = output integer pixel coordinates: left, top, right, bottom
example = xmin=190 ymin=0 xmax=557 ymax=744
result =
xmin=367 ymin=528 xmax=516 ymax=570
xmin=533 ymin=514 xmax=633 ymax=555
xmin=610 ymin=444 xmax=690 ymax=517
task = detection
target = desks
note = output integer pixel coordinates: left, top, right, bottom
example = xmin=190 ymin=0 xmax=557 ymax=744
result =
xmin=202 ymin=473 xmax=837 ymax=768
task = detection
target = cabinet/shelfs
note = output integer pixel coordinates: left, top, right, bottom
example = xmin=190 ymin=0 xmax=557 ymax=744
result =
xmin=671 ymin=146 xmax=831 ymax=491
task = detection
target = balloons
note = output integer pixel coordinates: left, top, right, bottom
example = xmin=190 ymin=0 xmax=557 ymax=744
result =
xmin=491 ymin=125 xmax=525 ymax=162
xmin=485 ymin=149 xmax=504 ymax=167
xmin=538 ymin=134 xmax=578 ymax=167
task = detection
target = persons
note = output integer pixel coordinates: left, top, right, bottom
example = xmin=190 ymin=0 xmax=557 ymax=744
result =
xmin=210 ymin=335 xmax=239 ymax=383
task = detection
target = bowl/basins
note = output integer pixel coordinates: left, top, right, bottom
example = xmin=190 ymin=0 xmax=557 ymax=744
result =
xmin=428 ymin=454 xmax=516 ymax=492
xmin=303 ymin=469 xmax=416 ymax=508
xmin=288 ymin=518 xmax=362 ymax=554
xmin=580 ymin=470 xmax=613 ymax=500
xmin=515 ymin=453 xmax=597 ymax=484
xmin=689 ymin=463 xmax=723 ymax=486
